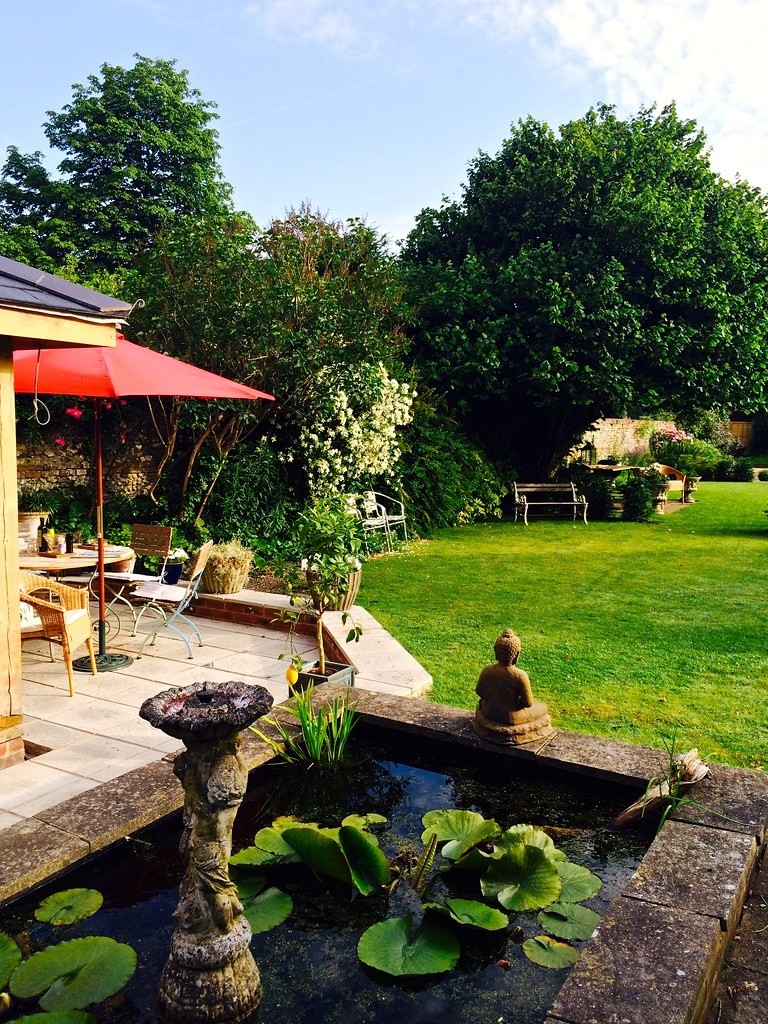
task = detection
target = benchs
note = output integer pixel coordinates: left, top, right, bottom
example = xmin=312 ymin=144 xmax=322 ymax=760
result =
xmin=510 ymin=481 xmax=589 ymax=526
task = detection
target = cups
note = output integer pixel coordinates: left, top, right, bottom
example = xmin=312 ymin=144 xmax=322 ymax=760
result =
xmin=27 ymin=543 xmax=39 ymax=557
xmin=66 ymin=534 xmax=74 ymax=553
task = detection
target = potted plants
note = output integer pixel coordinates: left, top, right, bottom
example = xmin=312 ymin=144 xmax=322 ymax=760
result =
xmin=662 ymin=437 xmax=722 ymax=503
xmin=193 ymin=532 xmax=256 ymax=596
xmin=288 ymin=495 xmax=370 ymax=611
xmin=18 ymin=485 xmax=61 ymax=552
xmin=270 ymin=565 xmax=364 ymax=699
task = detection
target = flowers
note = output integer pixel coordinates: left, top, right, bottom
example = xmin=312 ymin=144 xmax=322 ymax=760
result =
xmin=642 ymin=463 xmax=671 ymax=484
xmin=159 ymin=548 xmax=189 ymax=563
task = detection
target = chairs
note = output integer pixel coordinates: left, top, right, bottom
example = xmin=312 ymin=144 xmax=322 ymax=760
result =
xmin=660 ymin=465 xmax=686 ymax=505
xmin=19 ymin=571 xmax=98 ymax=698
xmin=129 ymin=539 xmax=214 ymax=659
xmin=339 ymin=491 xmax=408 ymax=559
xmin=93 ymin=523 xmax=172 ymax=637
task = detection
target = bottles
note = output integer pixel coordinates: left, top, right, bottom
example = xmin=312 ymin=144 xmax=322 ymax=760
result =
xmin=58 ymin=536 xmax=67 ymax=555
xmin=38 ymin=517 xmax=47 ymax=552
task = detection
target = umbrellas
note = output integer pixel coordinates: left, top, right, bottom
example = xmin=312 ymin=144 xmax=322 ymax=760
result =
xmin=13 ymin=331 xmax=278 ymax=658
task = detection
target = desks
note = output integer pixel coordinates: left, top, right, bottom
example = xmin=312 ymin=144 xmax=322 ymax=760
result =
xmin=586 ymin=465 xmax=640 ymax=486
xmin=19 ymin=543 xmax=134 ymax=643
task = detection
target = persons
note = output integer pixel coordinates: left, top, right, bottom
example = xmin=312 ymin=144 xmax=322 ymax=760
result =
xmin=476 ymin=629 xmax=549 ymax=727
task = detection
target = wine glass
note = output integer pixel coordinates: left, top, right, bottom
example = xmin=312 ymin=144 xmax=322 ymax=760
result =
xmin=47 ymin=537 xmax=55 ymax=553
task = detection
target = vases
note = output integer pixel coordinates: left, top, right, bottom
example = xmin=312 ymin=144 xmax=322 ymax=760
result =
xmin=650 ymin=484 xmax=672 ymax=515
xmin=156 ymin=562 xmax=184 ymax=584
